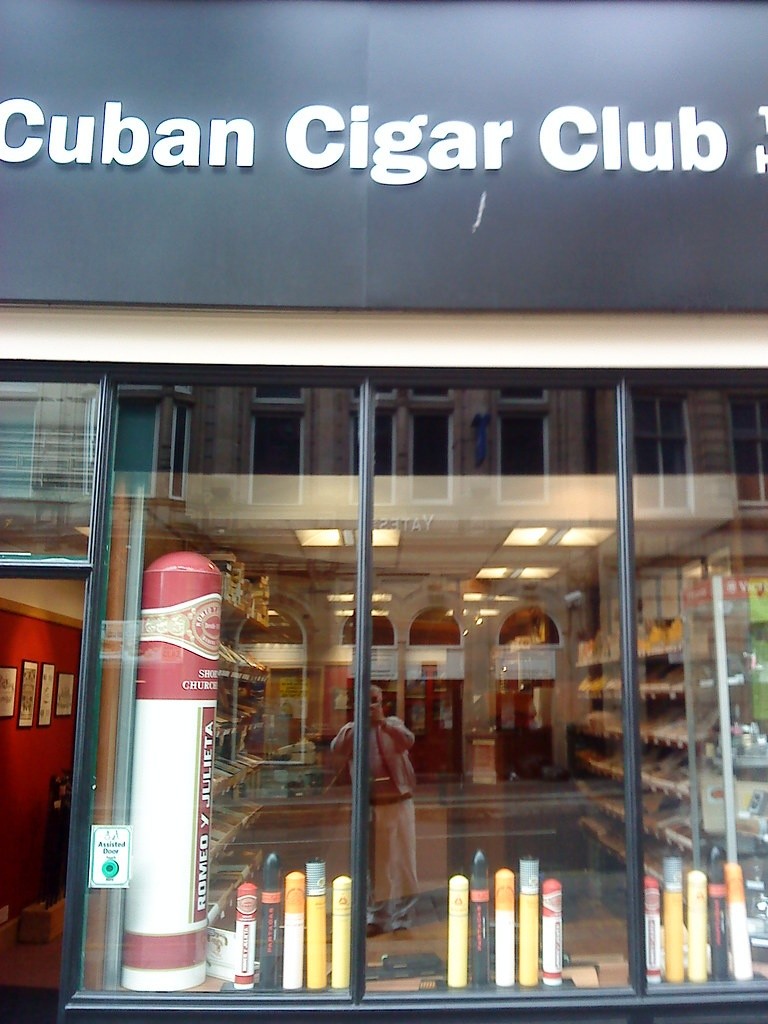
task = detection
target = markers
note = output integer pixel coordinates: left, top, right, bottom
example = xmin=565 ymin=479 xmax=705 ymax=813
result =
xmin=641 ymin=847 xmax=754 ymax=984
xmin=447 ymin=849 xmax=565 ymax=987
xmin=122 ymin=550 xmax=223 ymax=992
xmin=234 ymin=852 xmax=352 ymax=991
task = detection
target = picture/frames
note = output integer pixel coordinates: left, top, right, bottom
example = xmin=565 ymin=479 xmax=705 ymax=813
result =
xmin=55 ymin=672 xmax=74 ymax=718
xmin=0 ymin=666 xmax=17 ymax=718
xmin=16 ymin=660 xmax=38 ymax=729
xmin=37 ymin=662 xmax=54 ymax=727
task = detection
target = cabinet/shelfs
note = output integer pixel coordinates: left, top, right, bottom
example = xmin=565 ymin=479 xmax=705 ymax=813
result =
xmin=575 ymin=638 xmax=718 ymax=908
xmin=207 ymin=596 xmax=270 ymax=927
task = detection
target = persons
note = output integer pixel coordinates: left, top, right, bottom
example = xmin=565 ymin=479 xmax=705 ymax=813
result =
xmin=330 ymin=684 xmax=419 ymax=938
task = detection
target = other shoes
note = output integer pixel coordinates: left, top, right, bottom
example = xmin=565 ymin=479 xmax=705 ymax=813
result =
xmin=388 ymin=920 xmax=413 ymax=939
xmin=366 ymin=908 xmax=378 ymax=936
